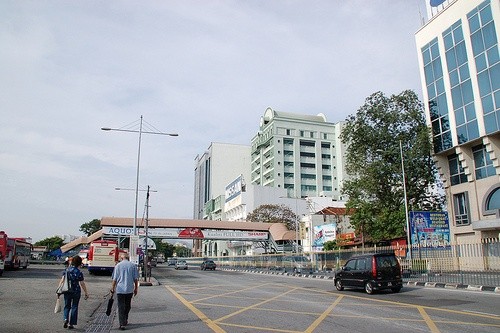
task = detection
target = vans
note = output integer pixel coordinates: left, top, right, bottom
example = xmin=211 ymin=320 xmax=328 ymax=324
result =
xmin=333 ymin=251 xmax=404 ymax=296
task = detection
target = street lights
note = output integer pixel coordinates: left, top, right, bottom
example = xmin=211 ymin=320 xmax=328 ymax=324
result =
xmin=279 ymin=195 xmax=299 ymax=253
xmin=99 ymin=114 xmax=179 ymax=236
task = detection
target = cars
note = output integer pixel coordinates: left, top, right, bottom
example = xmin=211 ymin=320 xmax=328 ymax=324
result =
xmin=200 ymin=260 xmax=216 ymax=270
xmin=150 ymin=256 xmax=177 ymax=267
xmin=174 ymin=259 xmax=188 ymax=270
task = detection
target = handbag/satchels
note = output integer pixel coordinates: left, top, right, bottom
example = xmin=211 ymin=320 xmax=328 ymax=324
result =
xmin=56 ymin=271 xmax=69 ymax=294
xmin=53 ymin=294 xmax=62 ymax=314
xmin=105 ymin=292 xmax=114 ymax=316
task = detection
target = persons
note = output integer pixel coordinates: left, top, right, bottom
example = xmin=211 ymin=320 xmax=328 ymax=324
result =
xmin=56 ymin=256 xmax=88 ymax=329
xmin=111 ymin=253 xmax=140 ymax=330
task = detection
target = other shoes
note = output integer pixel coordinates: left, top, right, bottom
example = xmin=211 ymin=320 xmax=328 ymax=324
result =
xmin=68 ymin=324 xmax=75 ymax=329
xmin=119 ymin=324 xmax=125 ymax=329
xmin=63 ymin=318 xmax=69 ymax=329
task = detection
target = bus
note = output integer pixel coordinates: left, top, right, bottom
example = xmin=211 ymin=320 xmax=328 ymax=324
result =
xmin=5 ymin=237 xmax=33 ymax=271
xmin=78 ymin=249 xmax=90 ymax=268
xmin=86 ymin=238 xmax=119 ymax=276
xmin=0 ymin=231 xmax=8 ymax=277
